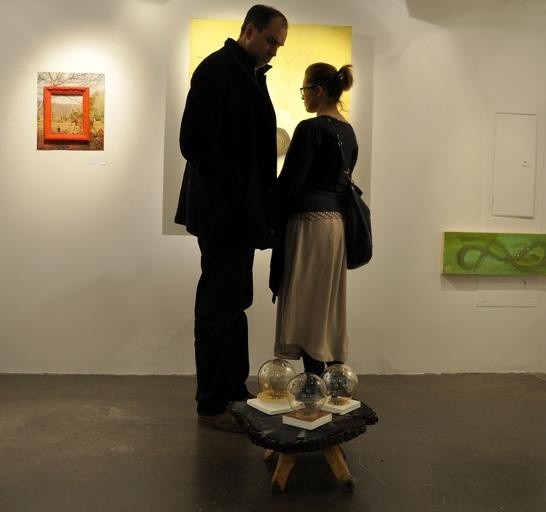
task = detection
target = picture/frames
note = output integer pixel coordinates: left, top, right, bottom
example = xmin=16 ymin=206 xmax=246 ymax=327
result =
xmin=43 ymin=86 xmax=86 ymax=140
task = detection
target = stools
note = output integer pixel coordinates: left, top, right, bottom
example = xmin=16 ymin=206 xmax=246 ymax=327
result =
xmin=239 ymin=395 xmax=378 ymax=494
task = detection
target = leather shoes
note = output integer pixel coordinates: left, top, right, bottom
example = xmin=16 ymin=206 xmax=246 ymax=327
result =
xmin=197 ymin=411 xmax=244 ymax=432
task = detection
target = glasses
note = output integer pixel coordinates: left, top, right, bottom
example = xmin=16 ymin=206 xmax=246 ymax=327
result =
xmin=300 ymin=85 xmax=325 ymax=95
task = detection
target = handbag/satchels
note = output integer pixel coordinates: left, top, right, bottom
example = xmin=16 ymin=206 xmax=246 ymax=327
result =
xmin=342 ymin=181 xmax=373 ymax=270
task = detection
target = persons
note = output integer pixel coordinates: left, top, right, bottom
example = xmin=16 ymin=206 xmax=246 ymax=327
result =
xmin=174 ymin=6 xmax=289 ymax=434
xmin=269 ymin=63 xmax=359 ymax=379
